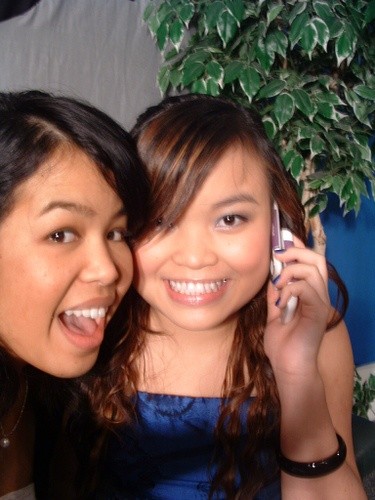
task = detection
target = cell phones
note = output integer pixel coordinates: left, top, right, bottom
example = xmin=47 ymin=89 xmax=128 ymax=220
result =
xmin=271 ymin=202 xmax=299 ymax=325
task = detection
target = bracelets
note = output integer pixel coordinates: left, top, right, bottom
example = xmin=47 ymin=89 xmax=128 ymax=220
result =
xmin=272 ymin=432 xmax=348 ymax=478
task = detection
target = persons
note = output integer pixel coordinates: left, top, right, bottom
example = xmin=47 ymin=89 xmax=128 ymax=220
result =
xmin=123 ymin=93 xmax=369 ymax=500
xmin=2 ymin=83 xmax=156 ymax=500
